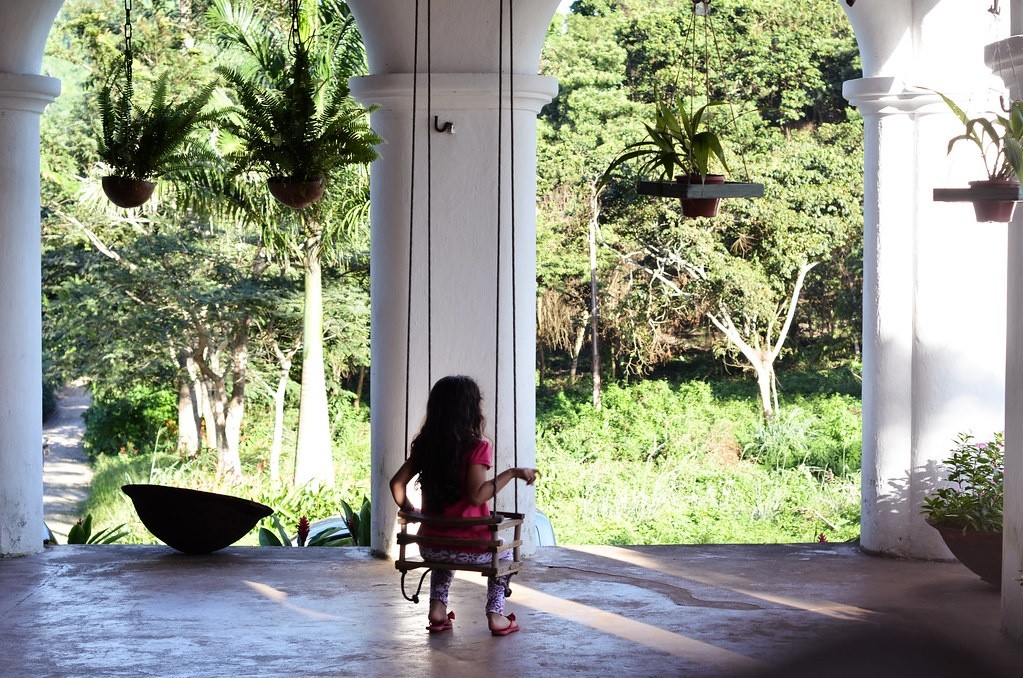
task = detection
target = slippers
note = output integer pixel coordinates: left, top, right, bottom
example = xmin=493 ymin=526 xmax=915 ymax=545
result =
xmin=491 ymin=613 xmax=519 ymax=636
xmin=426 ymin=611 xmax=455 ymax=631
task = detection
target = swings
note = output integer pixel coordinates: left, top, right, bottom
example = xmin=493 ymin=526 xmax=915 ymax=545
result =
xmin=396 ymin=0 xmax=525 ymax=603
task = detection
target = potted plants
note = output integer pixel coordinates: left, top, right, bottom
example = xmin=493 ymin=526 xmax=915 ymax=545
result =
xmin=913 ymin=85 xmax=1023 ymax=223
xmin=593 ymin=83 xmax=788 ymax=217
xmin=99 ymin=69 xmax=220 ymax=208
xmin=212 ymin=18 xmax=382 ymax=208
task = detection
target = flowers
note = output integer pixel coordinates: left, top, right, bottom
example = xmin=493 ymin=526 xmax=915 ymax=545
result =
xmin=918 ymin=432 xmax=1003 ymax=534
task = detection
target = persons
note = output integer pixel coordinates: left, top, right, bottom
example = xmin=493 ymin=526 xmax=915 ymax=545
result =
xmin=389 ymin=377 xmax=541 ymax=636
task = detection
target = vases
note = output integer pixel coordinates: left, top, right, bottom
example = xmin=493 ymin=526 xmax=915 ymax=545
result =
xmin=938 ymin=528 xmax=1002 ymax=582
